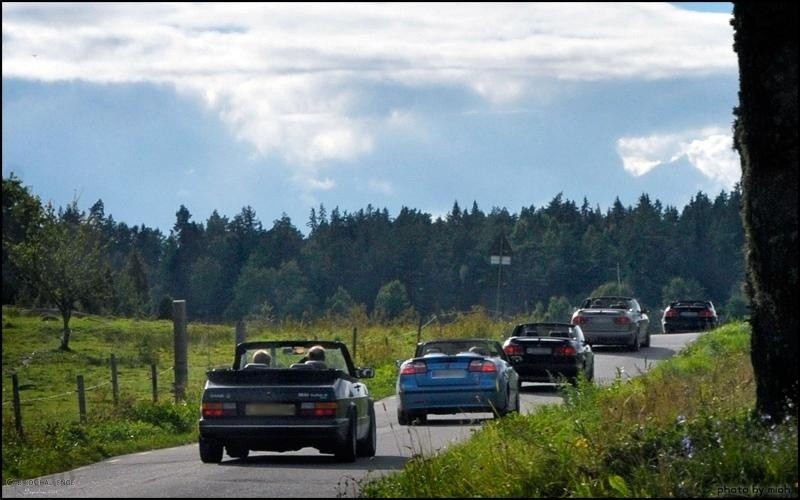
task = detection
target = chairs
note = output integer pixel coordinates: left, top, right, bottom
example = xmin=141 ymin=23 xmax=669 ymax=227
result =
xmin=243 ymin=363 xmax=268 ymax=369
xmin=677 ymin=303 xmax=686 ymax=306
xmin=469 ymin=346 xmax=491 ymax=357
xmin=424 ymin=347 xmax=441 ymax=353
xmin=549 ymin=330 xmax=569 ymax=337
xmin=693 ymin=303 xmax=705 ymax=306
xmin=525 ymin=330 xmax=538 ymax=336
xmin=591 ymin=305 xmax=602 ymax=308
xmin=612 ymin=305 xmax=622 ymax=309
xmin=306 ymin=360 xmax=327 ymax=371
xmin=289 ymin=362 xmax=314 ymax=369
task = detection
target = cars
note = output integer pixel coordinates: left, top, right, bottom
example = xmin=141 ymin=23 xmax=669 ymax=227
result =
xmin=572 ymin=295 xmax=651 ymax=351
xmin=395 ymin=336 xmax=522 ymax=422
xmin=505 ymin=322 xmax=596 ymax=390
xmin=196 ymin=336 xmax=382 ymax=465
xmin=659 ymin=298 xmax=720 ymax=334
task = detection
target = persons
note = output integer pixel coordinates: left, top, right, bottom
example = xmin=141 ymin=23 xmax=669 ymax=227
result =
xmin=252 ymin=350 xmax=271 ymax=368
xmin=298 ymin=345 xmax=327 ymax=369
xmin=595 ymin=299 xmax=603 ymax=305
xmin=616 ymin=301 xmax=626 ymax=306
